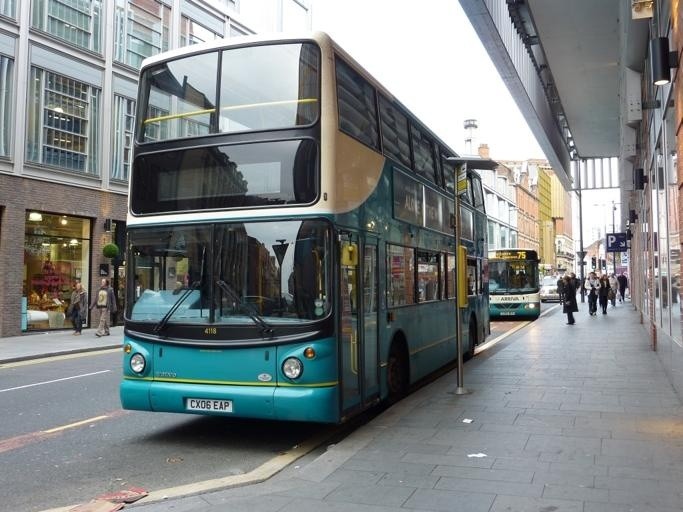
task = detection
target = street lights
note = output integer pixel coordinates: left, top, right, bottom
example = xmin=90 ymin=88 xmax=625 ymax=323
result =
xmin=590 ymin=199 xmax=615 ymax=275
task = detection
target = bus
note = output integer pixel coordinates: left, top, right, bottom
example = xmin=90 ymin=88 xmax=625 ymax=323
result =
xmin=487 ymin=247 xmax=544 ymax=323
xmin=112 ymin=30 xmax=494 ymax=431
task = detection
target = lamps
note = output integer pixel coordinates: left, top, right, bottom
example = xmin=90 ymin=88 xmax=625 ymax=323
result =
xmin=628 ymin=209 xmax=638 ymax=224
xmin=649 ymin=36 xmax=679 ymax=87
xmin=625 ymin=229 xmax=632 ymax=240
xmin=634 ymin=167 xmax=649 ymax=192
xmin=102 ymin=218 xmax=112 ymax=234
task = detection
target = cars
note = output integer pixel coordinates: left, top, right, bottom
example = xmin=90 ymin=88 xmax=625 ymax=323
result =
xmin=540 ymin=276 xmax=553 ymax=285
xmin=539 ymin=278 xmax=564 ymax=302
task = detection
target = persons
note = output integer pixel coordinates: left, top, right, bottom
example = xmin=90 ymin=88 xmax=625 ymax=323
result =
xmin=655 ymin=274 xmax=680 ymax=310
xmin=89 ymin=278 xmax=117 ymax=338
xmin=68 ymin=283 xmax=87 ymax=336
xmin=557 ymin=271 xmax=628 ymax=325
xmin=508 ymin=266 xmax=530 ymax=288
xmin=468 ymin=273 xmax=474 ymax=295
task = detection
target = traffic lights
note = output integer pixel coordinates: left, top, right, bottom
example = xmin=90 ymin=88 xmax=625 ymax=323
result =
xmin=591 ymin=256 xmax=596 ymax=269
xmin=602 ymin=259 xmax=606 ymax=270
